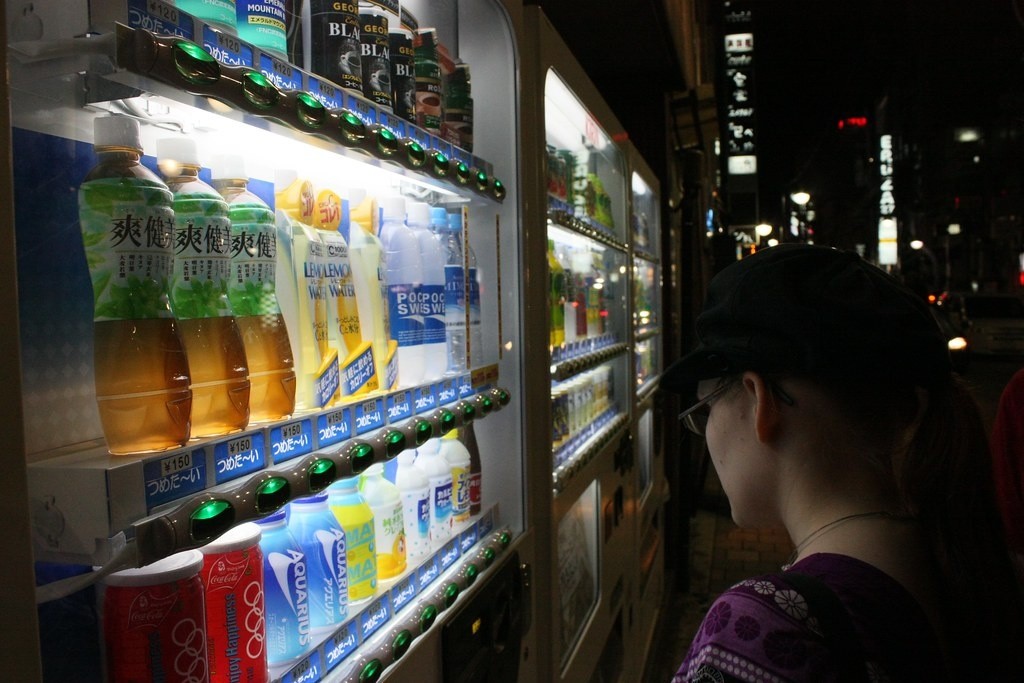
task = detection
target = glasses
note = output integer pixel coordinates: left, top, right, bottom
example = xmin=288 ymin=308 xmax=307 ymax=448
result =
xmin=679 ymin=378 xmax=740 ymax=441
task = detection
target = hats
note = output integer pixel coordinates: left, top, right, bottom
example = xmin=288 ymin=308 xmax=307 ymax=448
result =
xmin=655 ymin=244 xmax=953 ymax=413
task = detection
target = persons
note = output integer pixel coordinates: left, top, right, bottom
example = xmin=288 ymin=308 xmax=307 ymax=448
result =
xmin=945 ymin=244 xmax=973 ymax=331
xmin=990 ymin=368 xmax=1024 ymax=593
xmin=660 ymin=244 xmax=985 ymax=683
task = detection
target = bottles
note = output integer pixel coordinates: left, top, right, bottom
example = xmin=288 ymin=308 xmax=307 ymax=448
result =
xmin=208 ymin=159 xmax=297 ymax=424
xmin=547 ymin=145 xmax=615 ymax=342
xmin=252 ymin=421 xmax=481 ymax=666
xmin=77 ymin=115 xmax=194 ymax=457
xmin=379 ymin=196 xmax=484 ymax=387
xmin=155 ymin=137 xmax=250 ymax=440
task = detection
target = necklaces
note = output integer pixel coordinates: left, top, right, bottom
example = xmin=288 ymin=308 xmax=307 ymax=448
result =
xmin=782 ymin=512 xmax=909 ymax=570
